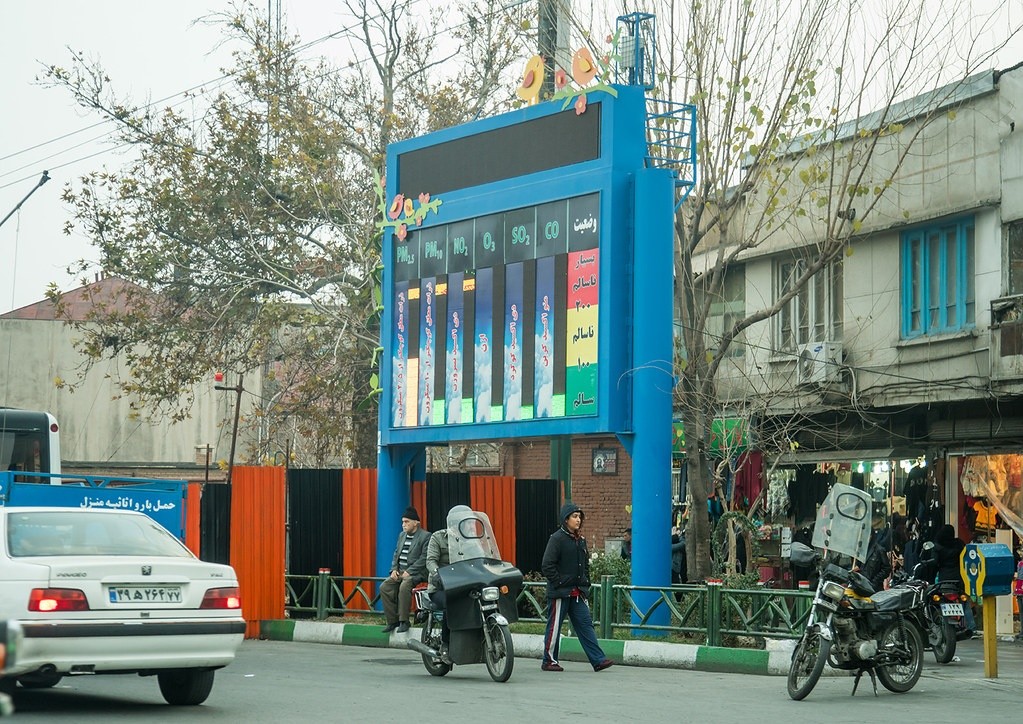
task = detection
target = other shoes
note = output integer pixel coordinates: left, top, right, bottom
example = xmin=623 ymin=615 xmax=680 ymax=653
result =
xmin=1016 ymin=633 xmax=1023 ymax=638
xmin=383 ymin=621 xmax=400 ymax=633
xmin=923 ymin=643 xmax=933 ymax=651
xmin=439 ymin=645 xmax=452 ymax=664
xmin=541 ymin=664 xmax=563 ymax=671
xmin=397 ymin=621 xmax=411 ymax=633
xmin=971 ymin=631 xmax=982 ymax=640
xmin=594 ymin=660 xmax=613 ymax=672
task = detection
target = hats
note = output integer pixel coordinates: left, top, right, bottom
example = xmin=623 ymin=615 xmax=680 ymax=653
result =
xmin=402 ymin=506 xmax=420 ymax=521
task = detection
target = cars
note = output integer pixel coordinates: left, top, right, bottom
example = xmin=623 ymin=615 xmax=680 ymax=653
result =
xmin=0 ymin=503 xmax=247 ymax=706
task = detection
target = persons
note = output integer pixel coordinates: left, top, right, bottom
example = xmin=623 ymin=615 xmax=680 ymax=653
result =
xmin=933 ymin=525 xmax=976 ymax=634
xmin=671 ymin=534 xmax=684 ymax=602
xmin=540 ymin=501 xmax=614 ymax=671
xmin=379 ymin=507 xmax=433 ymax=632
xmin=620 ymin=529 xmax=631 ymax=561
xmin=860 ymin=527 xmax=891 ymax=592
xmin=426 ymin=526 xmax=484 ymax=666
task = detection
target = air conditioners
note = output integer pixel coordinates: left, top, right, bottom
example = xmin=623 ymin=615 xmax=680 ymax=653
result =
xmin=796 ymin=341 xmax=842 ymax=384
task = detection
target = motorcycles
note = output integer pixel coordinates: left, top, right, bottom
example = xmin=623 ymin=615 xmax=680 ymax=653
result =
xmin=785 ymin=481 xmax=978 ymax=701
xmin=406 ymin=510 xmax=524 ymax=683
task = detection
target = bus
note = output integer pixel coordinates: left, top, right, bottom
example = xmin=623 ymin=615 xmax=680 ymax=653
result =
xmin=0 ymin=406 xmax=63 ymax=485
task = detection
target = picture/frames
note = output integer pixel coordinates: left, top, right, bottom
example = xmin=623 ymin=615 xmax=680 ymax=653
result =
xmin=592 ymin=447 xmax=619 ymax=476
